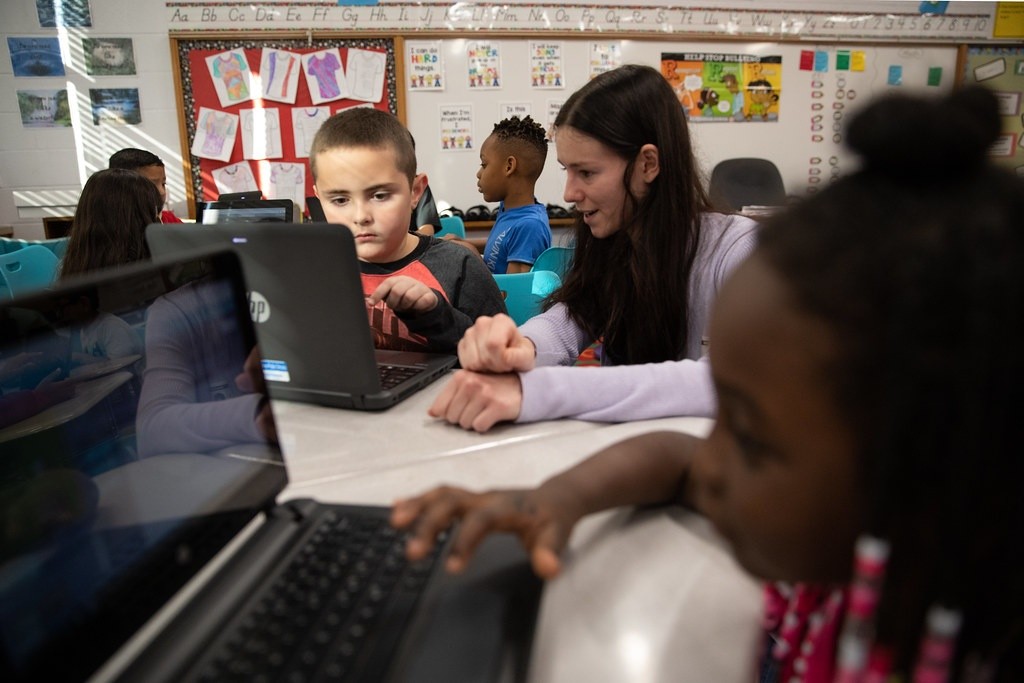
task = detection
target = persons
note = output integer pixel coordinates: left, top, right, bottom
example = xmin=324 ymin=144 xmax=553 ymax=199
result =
xmin=312 ymin=108 xmax=509 ymax=357
xmin=476 ymin=116 xmax=552 ymax=274
xmin=109 ymin=148 xmax=184 ymax=223
xmin=430 ymin=64 xmax=765 ymax=432
xmin=412 ymin=174 xmax=443 ymax=237
xmin=133 ymin=259 xmax=273 ymax=457
xmin=0 ymin=313 xmax=142 ymax=423
xmin=390 ymin=161 xmax=1024 ymax=683
xmin=56 ymin=168 xmax=160 ymax=277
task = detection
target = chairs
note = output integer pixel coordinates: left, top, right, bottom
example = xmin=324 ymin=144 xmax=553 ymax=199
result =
xmin=0 ymin=245 xmax=60 ymax=298
xmin=708 ymin=158 xmax=787 ymax=211
xmin=492 ymin=270 xmax=563 ymax=326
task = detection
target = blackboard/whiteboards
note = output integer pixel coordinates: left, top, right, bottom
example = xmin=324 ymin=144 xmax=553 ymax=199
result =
xmin=397 ymin=32 xmax=964 ymax=228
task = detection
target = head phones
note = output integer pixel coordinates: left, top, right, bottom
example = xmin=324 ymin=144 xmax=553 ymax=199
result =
xmin=437 ymin=203 xmax=583 ymax=222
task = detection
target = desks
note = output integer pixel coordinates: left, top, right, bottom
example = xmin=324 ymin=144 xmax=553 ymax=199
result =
xmin=271 ymin=369 xmax=611 ymax=486
xmin=276 ymin=417 xmax=715 ymax=550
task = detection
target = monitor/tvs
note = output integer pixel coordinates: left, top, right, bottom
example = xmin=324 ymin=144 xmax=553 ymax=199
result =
xmin=194 ymin=197 xmax=295 ymax=225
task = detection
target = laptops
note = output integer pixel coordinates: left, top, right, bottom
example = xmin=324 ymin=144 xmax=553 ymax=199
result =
xmin=0 ymin=243 xmax=543 ymax=683
xmin=18 ymin=325 xmax=73 ymax=403
xmin=144 ymin=222 xmax=460 ymax=413
